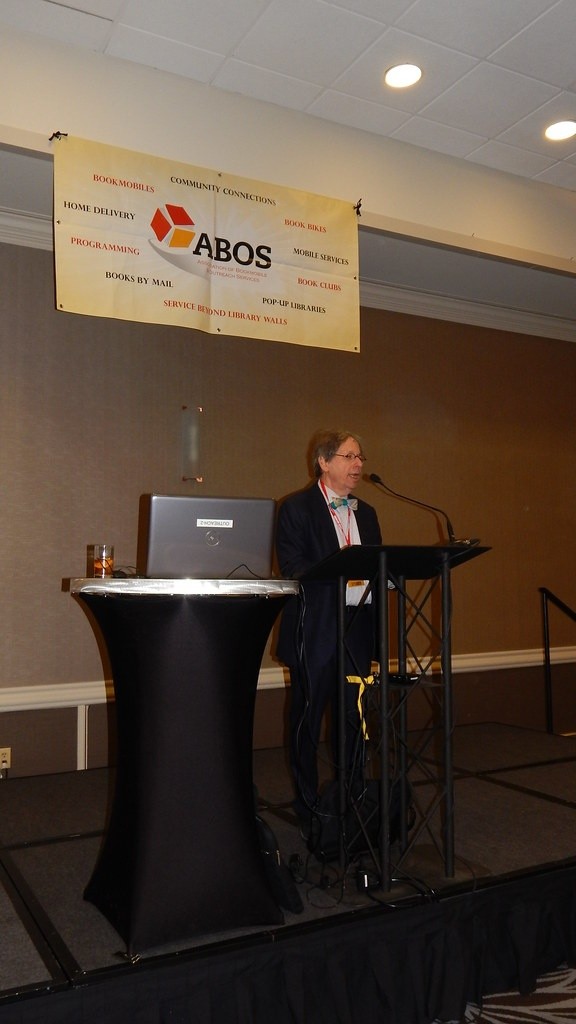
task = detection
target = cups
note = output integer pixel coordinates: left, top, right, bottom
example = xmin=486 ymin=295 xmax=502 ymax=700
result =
xmin=94 ymin=545 xmax=114 ymax=579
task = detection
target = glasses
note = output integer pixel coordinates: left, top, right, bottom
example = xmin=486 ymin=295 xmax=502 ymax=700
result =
xmin=333 ymin=452 xmax=367 ymax=462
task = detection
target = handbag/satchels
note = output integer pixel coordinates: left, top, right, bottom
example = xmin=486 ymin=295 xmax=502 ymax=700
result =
xmin=311 ymin=767 xmax=419 ymax=864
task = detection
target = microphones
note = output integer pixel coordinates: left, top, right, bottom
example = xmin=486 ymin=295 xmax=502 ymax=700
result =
xmin=370 ymin=473 xmax=457 ymax=544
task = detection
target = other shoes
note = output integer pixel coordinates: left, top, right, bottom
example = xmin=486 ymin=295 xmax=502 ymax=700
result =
xmin=297 ymin=810 xmax=322 ymax=843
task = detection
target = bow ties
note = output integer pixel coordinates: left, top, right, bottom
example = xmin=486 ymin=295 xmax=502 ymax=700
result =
xmin=330 ymin=496 xmax=358 ymax=511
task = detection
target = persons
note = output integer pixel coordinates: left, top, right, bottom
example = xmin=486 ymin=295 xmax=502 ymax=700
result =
xmin=275 ymin=429 xmax=385 ymax=842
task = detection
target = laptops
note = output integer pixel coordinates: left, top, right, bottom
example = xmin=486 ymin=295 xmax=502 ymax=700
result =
xmin=125 ymin=493 xmax=276 ymax=579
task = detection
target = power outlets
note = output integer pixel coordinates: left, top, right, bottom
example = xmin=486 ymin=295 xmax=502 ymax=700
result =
xmin=0 ymin=748 xmax=12 ymax=770
